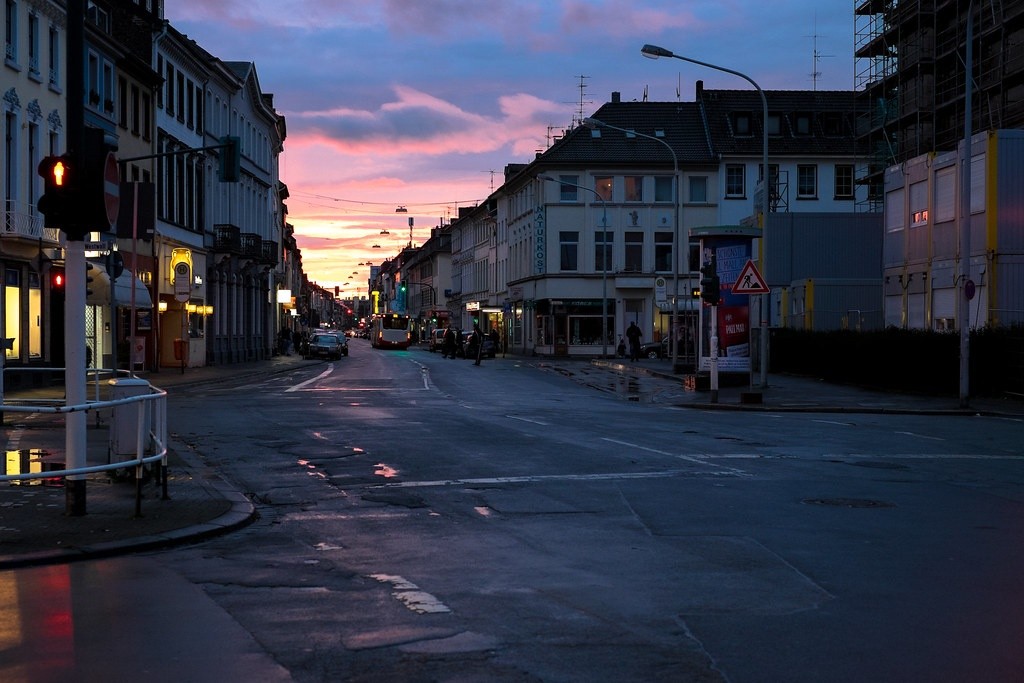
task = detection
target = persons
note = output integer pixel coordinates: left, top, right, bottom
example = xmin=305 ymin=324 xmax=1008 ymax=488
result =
xmin=277 ymin=324 xmax=302 ymax=356
xmin=455 ymin=328 xmax=466 ymax=360
xmin=626 ymin=321 xmax=643 ymax=361
xmin=491 ymin=329 xmax=500 ymax=354
xmin=442 ymin=328 xmax=455 ymax=360
xmin=472 ymin=327 xmax=484 ymax=367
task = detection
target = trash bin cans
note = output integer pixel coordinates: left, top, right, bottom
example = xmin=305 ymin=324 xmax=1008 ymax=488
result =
xmin=174 ymin=338 xmax=187 ymax=360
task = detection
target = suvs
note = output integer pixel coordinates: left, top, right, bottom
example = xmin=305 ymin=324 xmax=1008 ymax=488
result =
xmin=429 ymin=328 xmax=497 ymax=359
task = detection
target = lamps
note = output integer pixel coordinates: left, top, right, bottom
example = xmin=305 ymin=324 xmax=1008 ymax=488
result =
xmin=206 ymin=306 xmax=213 ymax=316
xmin=629 ymin=211 xmax=638 ymax=225
xmin=185 ymin=304 xmax=197 ymax=313
xmin=377 ymin=282 xmax=381 ymax=285
xmin=158 ymin=299 xmax=167 ymax=315
xmin=401 ymin=259 xmax=407 ymax=265
xmin=142 ymin=271 xmax=152 ymax=285
xmin=197 ymin=305 xmax=204 ymax=315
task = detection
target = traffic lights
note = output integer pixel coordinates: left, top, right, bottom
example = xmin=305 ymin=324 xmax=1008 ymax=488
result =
xmin=347 ymin=308 xmax=352 ymax=315
xmin=700 ymin=255 xmax=717 ymax=278
xmin=37 ymin=152 xmax=67 ymax=214
xmin=50 ymin=267 xmax=65 ymax=290
xmin=692 ymin=288 xmax=700 ymax=299
xmin=400 ymin=280 xmax=406 ymax=293
xmin=432 ymin=315 xmax=438 ymax=324
xmin=474 ymin=316 xmax=479 ymax=327
xmin=700 ymin=276 xmax=720 ymax=306
xmin=85 ymin=261 xmax=94 ymax=299
xmin=43 ymin=152 xmax=94 ymax=230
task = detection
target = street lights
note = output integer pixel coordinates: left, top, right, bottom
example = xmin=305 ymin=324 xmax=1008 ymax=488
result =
xmin=583 ymin=117 xmax=680 ymax=370
xmin=537 ymin=174 xmax=607 ymax=359
xmin=641 ymin=44 xmax=769 ymax=391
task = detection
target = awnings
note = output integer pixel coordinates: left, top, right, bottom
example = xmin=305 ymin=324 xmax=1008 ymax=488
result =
xmin=86 ymin=260 xmax=152 ymax=309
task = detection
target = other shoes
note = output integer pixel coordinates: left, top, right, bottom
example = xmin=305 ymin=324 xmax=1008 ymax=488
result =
xmin=472 ymin=362 xmax=481 ymax=366
xmin=630 ymin=360 xmax=640 ymax=362
xmin=449 ymin=357 xmax=455 ymax=359
xmin=441 ymin=356 xmax=447 ymax=359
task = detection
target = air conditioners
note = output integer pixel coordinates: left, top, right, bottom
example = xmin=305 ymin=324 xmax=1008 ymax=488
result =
xmin=240 ymin=237 xmax=260 ymax=255
xmin=257 ymin=243 xmax=276 ymax=262
xmin=217 ymin=228 xmax=238 ymax=249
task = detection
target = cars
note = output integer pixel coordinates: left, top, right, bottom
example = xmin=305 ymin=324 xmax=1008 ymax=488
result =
xmin=307 ymin=333 xmax=343 ymax=361
xmin=297 ymin=326 xmax=372 ymax=356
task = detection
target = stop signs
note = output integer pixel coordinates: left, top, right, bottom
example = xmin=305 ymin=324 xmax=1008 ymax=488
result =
xmin=103 ymin=151 xmax=121 ymax=226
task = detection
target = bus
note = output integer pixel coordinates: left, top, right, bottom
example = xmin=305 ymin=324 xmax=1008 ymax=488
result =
xmin=368 ymin=312 xmax=412 ymax=349
